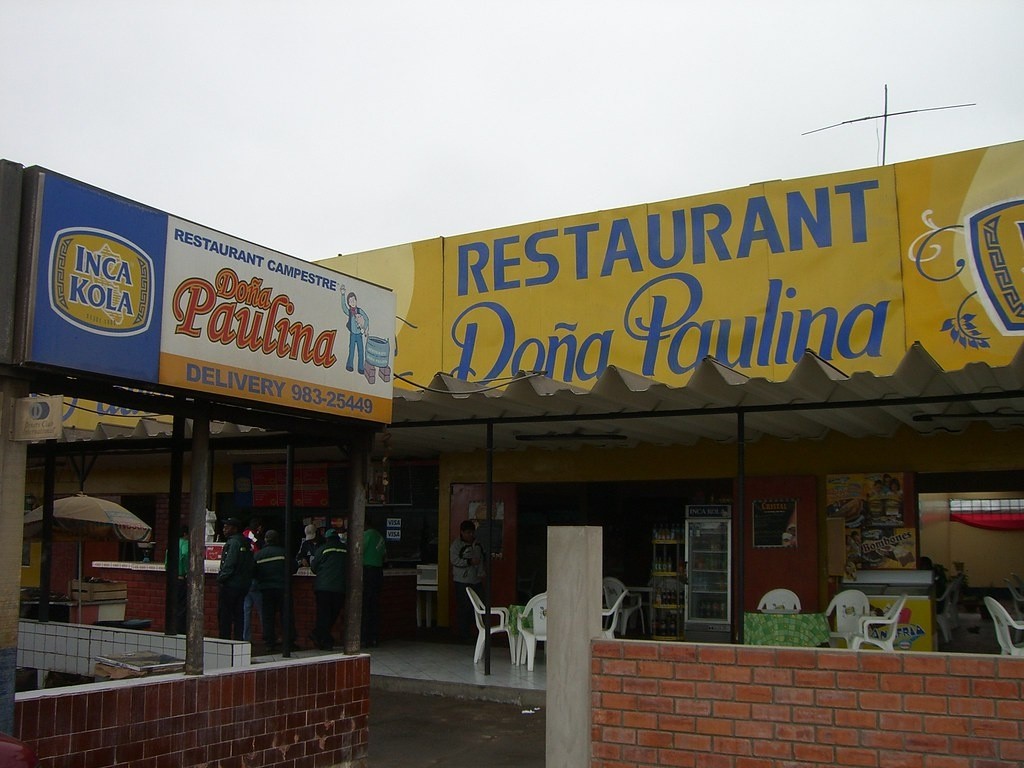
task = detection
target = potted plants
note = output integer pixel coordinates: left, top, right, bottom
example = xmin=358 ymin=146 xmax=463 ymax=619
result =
xmin=67 ymin=575 xmax=128 ymax=602
xmin=949 ymin=560 xmax=977 ymax=611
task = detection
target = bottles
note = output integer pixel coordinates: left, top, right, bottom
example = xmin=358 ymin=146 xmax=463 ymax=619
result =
xmin=689 ymin=523 xmax=728 ymax=619
xmin=649 ymin=522 xmax=684 ymax=637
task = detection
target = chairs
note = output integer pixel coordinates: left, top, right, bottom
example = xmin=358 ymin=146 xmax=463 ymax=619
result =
xmin=466 ymin=587 xmax=548 ymax=671
xmin=602 ymin=577 xmax=645 ymax=640
xmin=935 ymin=578 xmax=961 ymax=644
xmin=823 ymin=588 xmax=908 ymax=651
xmin=517 ymin=575 xmax=536 ymax=597
xmin=756 ymin=588 xmax=801 ymax=610
xmin=984 ymin=571 xmax=1024 ymax=656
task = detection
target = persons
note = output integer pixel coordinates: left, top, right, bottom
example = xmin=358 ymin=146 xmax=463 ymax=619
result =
xmin=164 ymin=525 xmax=190 ymax=633
xmin=363 ymin=522 xmax=387 ymax=648
xmin=306 ymin=529 xmax=347 ymax=650
xmin=216 ymin=517 xmax=298 ymax=650
xmin=295 ymin=523 xmax=325 ymax=569
xmin=450 ymin=520 xmax=488 ymax=643
xmin=867 ymin=474 xmax=903 ymax=496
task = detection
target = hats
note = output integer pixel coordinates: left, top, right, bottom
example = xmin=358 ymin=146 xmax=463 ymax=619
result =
xmin=219 ymin=516 xmax=240 ymax=528
xmin=265 ymin=529 xmax=279 ymax=541
xmin=325 ymin=530 xmax=337 ymax=538
xmin=305 ymin=525 xmax=317 ymax=541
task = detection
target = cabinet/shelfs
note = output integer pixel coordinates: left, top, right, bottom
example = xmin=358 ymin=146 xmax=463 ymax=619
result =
xmin=650 ymin=536 xmax=685 ymax=641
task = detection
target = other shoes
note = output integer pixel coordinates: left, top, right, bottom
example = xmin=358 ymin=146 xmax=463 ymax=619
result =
xmin=310 ymin=634 xmax=323 ymax=647
xmin=265 ymin=638 xmax=282 ymax=646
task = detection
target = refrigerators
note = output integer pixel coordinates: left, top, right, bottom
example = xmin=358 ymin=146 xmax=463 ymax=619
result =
xmin=684 ymin=503 xmax=732 ymax=631
xmin=834 ymin=569 xmax=938 ymax=651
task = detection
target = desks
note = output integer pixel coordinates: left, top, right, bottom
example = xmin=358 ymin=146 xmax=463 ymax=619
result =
xmin=21 ymin=599 xmax=129 ymax=625
xmin=735 ymin=609 xmax=831 ymax=648
xmin=508 ymin=605 xmax=548 ymax=665
xmin=625 ymin=587 xmax=653 ymax=630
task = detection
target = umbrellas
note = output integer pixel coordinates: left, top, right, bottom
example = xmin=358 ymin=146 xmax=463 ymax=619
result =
xmin=22 ymin=492 xmax=153 ymax=624
